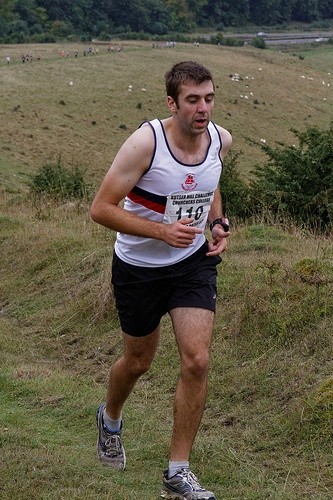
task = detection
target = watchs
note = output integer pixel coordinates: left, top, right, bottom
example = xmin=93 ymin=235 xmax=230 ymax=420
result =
xmin=208 ymin=216 xmax=230 ymax=232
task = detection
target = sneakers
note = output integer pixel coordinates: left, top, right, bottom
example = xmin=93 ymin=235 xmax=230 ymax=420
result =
xmin=160 ymin=466 xmax=214 ymax=500
xmin=96 ymin=404 xmax=126 ymax=472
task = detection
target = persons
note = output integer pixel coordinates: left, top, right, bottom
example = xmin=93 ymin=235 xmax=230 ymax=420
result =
xmin=89 ymin=60 xmax=233 ymax=500
